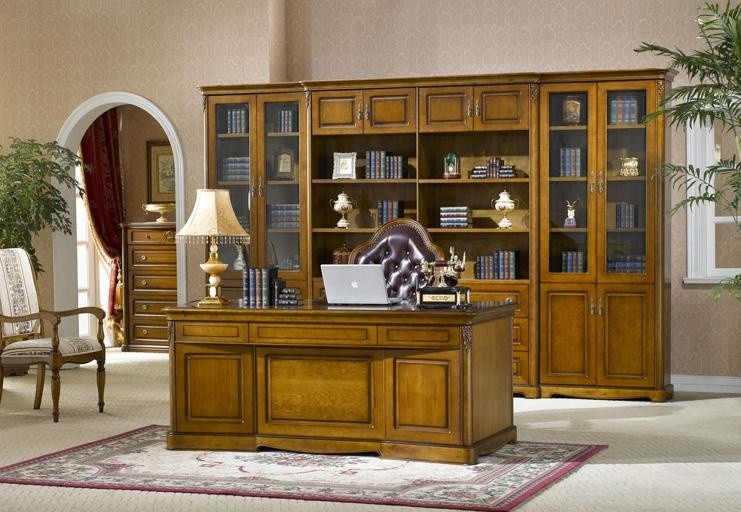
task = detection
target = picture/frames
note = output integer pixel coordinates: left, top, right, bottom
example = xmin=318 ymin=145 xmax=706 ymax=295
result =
xmin=146 ymin=141 xmax=176 ymax=204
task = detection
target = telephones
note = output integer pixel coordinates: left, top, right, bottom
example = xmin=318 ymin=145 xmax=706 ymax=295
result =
xmin=419 ymin=256 xmax=466 ymax=288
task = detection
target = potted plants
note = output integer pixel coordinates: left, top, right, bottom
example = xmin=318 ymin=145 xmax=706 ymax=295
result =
xmin=0 ymin=133 xmax=87 ymax=376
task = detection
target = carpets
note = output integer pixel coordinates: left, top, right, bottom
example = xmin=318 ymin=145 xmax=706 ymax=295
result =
xmin=0 ymin=423 xmax=608 ymax=512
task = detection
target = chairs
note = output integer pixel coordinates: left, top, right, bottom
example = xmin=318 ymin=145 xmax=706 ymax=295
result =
xmin=349 ymin=219 xmax=445 ymax=304
xmin=0 ymin=248 xmax=106 ymax=421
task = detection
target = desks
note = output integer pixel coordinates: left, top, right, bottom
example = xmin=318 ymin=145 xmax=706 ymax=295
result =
xmin=158 ymin=302 xmax=523 ymax=466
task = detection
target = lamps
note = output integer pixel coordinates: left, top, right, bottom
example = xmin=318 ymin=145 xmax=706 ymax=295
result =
xmin=174 ymin=188 xmax=251 ymax=307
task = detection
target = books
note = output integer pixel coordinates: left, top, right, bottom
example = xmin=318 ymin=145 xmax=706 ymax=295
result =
xmin=474 ymin=248 xmax=519 ymax=281
xmin=219 ymin=109 xmax=302 ymax=231
xmin=559 ymin=146 xmax=582 ymax=178
xmin=365 ymin=148 xmax=410 ymax=230
xmin=438 ymin=205 xmax=474 ymax=229
xmin=609 ymin=98 xmax=639 ymax=124
xmin=607 ymin=201 xmax=647 ymax=274
xmin=470 ymin=156 xmax=516 ymax=180
xmin=561 ymin=250 xmax=587 ymax=274
xmin=242 ymin=266 xmax=303 ymax=306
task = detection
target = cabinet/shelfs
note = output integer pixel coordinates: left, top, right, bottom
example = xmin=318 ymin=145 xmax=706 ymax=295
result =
xmin=539 ymin=68 xmax=679 ymax=403
xmin=302 ymin=72 xmax=540 ymax=399
xmin=197 ymin=82 xmax=307 ymax=299
xmin=120 ymin=221 xmax=177 ymax=352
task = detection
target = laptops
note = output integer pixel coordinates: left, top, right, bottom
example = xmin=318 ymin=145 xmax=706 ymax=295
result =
xmin=319 ymin=263 xmax=400 ymax=306
